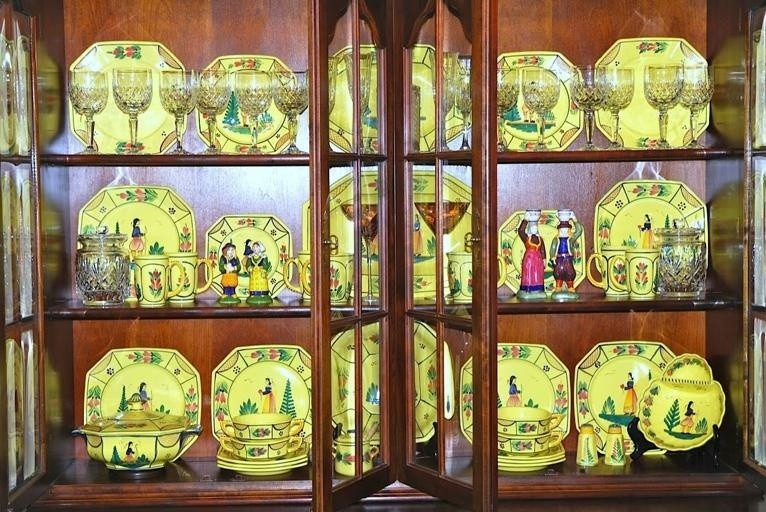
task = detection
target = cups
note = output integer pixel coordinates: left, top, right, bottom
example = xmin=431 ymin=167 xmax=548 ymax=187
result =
xmin=444 ymin=250 xmax=507 ymax=304
xmin=216 ymin=436 xmax=303 ymax=461
xmin=283 ymin=250 xmax=312 ymax=302
xmin=220 ymin=412 xmax=307 ymax=441
xmin=133 ymin=254 xmax=185 ymax=308
xmin=610 ymin=249 xmax=659 ymax=300
xmin=166 ymin=252 xmax=214 ymax=303
xmin=497 ymin=406 xmax=564 ymax=436
xmin=586 ymin=247 xmax=630 ymax=297
xmin=301 ymin=253 xmax=355 ymax=306
xmin=497 ymin=427 xmax=561 ymax=454
xmin=333 ymin=428 xmax=381 ymax=476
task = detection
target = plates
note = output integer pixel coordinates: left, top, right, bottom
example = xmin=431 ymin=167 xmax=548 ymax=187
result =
xmin=594 ymin=37 xmax=710 ymax=150
xmin=329 ymin=44 xmax=472 ymax=153
xmin=301 ymin=170 xmax=472 ymax=299
xmin=497 ymin=209 xmax=586 ymax=297
xmin=204 ymin=214 xmax=293 ymax=301
xmin=459 ymin=341 xmax=572 ymax=446
xmin=573 ymin=340 xmax=677 ymax=456
xmin=497 ymin=442 xmax=568 ymax=473
xmin=216 ymin=443 xmax=309 ymax=477
xmin=593 ymin=180 xmax=709 ymax=294
xmin=330 ymin=317 xmax=438 ymax=446
xmin=195 ymin=54 xmax=298 ymax=155
xmin=76 ymin=185 xmax=197 ymax=303
xmin=497 ymin=50 xmax=585 ymax=152
xmin=67 ymin=40 xmax=187 ymax=155
xmin=83 ymin=347 xmax=202 ymax=464
xmin=210 ymin=344 xmax=312 ymax=455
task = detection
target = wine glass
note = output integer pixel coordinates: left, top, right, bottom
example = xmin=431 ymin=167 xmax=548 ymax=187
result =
xmin=270 ymin=70 xmax=310 ymax=155
xmin=609 ymin=67 xmax=634 ymax=151
xmin=159 ymin=70 xmax=200 ymax=156
xmin=67 ymin=70 xmax=109 ymax=156
xmin=339 ymin=202 xmax=379 ymax=303
xmin=521 ymin=69 xmax=561 ymax=152
xmin=112 ymin=68 xmax=153 ymax=154
xmin=327 ymin=56 xmax=340 ymax=154
xmin=196 ymin=68 xmax=232 ymax=156
xmin=343 ymin=53 xmax=375 ymax=155
xmin=686 ymin=66 xmax=716 ymax=149
xmin=234 ymin=69 xmax=273 ymax=155
xmin=642 ymin=64 xmax=684 ymax=150
xmin=569 ymin=65 xmax=608 ymax=151
xmin=428 ymin=51 xmax=458 ymax=153
xmin=496 ymin=67 xmax=518 ymax=154
xmin=459 ymin=56 xmax=473 ymax=152
xmin=413 ymin=201 xmax=472 ymax=302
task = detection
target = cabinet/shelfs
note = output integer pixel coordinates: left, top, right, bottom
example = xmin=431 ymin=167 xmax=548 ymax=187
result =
xmin=0 ymin=1 xmax=766 ymax=510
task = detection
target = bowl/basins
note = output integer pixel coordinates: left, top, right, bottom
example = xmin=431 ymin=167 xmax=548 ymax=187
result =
xmin=556 ymin=208 xmax=574 ymax=222
xmin=526 ymin=209 xmax=541 ymax=223
xmin=636 ymin=352 xmax=726 ymax=453
